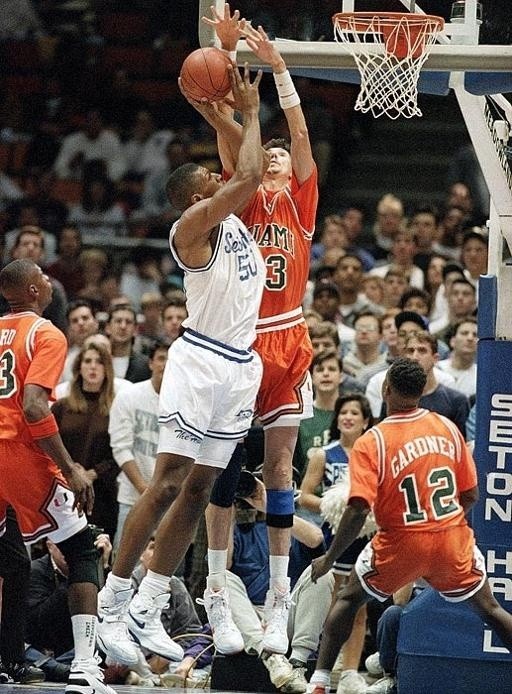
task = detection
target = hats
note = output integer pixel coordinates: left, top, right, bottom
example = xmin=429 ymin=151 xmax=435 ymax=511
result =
xmin=314 ymin=282 xmax=340 ymax=300
xmin=393 ymin=309 xmax=429 ymax=329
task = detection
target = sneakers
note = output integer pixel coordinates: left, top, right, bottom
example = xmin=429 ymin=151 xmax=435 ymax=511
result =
xmin=263 ymin=588 xmax=292 ymax=653
xmin=365 ymin=651 xmax=384 ymax=675
xmin=129 ymin=593 xmax=185 ymax=661
xmin=366 ymin=677 xmax=394 ymax=694
xmin=305 ymin=671 xmax=331 ymax=694
xmin=0 ymin=659 xmax=46 ymax=684
xmin=162 ymin=668 xmax=214 ymax=689
xmin=66 ymin=654 xmax=118 ymax=694
xmin=127 ymin=670 xmax=161 ymax=687
xmin=335 ymin=670 xmax=370 ymax=693
xmin=287 ymin=666 xmax=307 ymax=693
xmin=260 ymin=651 xmax=295 ymax=688
xmin=95 ymin=588 xmax=140 ymax=666
xmin=194 ymin=586 xmax=245 ymax=657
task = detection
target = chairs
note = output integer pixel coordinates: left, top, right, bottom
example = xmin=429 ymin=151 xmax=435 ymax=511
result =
xmin=1 ymin=0 xmax=352 ymax=318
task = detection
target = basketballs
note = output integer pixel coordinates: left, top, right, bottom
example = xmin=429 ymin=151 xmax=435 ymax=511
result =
xmin=180 ymin=48 xmax=237 ymax=102
